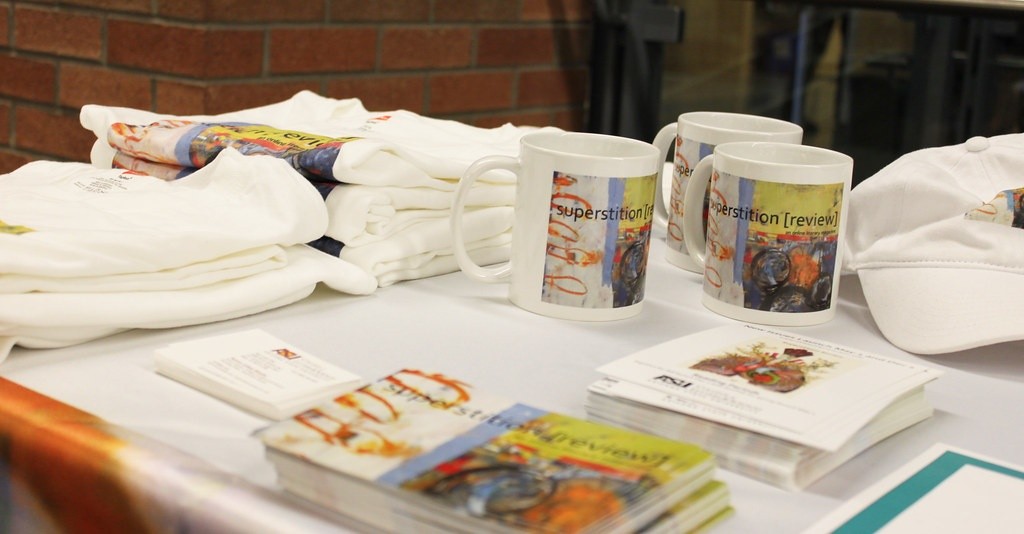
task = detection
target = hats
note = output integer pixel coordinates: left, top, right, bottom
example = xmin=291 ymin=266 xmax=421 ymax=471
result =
xmin=839 ymin=132 xmax=1024 ymax=354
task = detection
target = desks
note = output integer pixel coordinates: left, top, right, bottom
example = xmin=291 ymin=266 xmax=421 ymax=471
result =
xmin=0 ymin=255 xmax=1024 ymax=534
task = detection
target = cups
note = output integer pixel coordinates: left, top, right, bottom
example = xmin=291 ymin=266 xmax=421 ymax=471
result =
xmin=449 ymin=130 xmax=662 ymax=321
xmin=681 ymin=141 xmax=854 ymax=325
xmin=652 ymin=112 xmax=802 ymax=275
